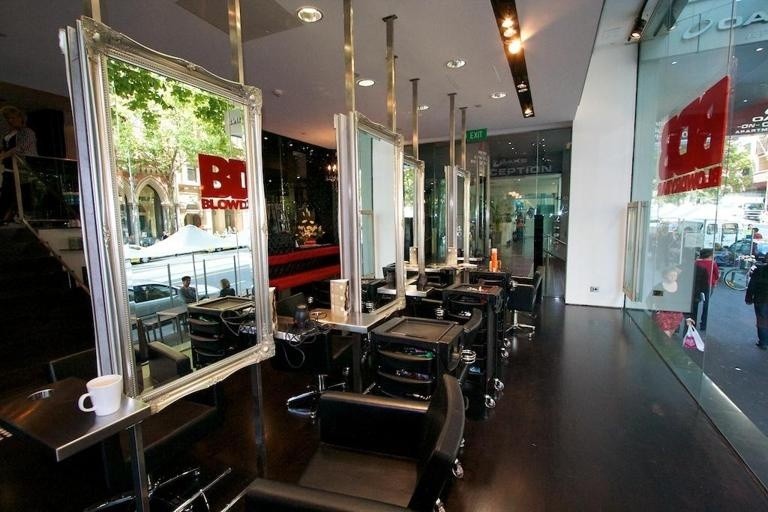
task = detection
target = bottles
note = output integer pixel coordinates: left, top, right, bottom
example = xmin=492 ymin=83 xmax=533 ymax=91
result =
xmin=293 ymin=304 xmax=310 ymax=327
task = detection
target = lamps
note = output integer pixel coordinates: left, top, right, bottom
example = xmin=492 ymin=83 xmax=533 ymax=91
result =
xmin=631 ymin=17 xmax=646 ymax=41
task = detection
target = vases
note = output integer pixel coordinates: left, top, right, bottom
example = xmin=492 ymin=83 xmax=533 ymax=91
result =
xmin=305 ymin=237 xmax=316 ymax=246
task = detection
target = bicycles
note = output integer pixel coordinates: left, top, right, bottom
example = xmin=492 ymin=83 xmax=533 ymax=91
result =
xmin=720 ymin=254 xmax=760 ymax=290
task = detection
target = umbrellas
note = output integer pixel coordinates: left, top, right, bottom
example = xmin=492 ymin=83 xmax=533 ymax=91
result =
xmin=126 ymin=223 xmax=233 ymax=298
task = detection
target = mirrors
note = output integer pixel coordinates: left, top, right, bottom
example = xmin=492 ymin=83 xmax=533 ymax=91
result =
xmin=334 ymin=110 xmax=408 ymax=313
xmin=443 ymin=164 xmax=471 ymax=265
xmin=59 ymin=16 xmax=275 ymax=425
xmin=404 ymin=152 xmax=425 ymax=285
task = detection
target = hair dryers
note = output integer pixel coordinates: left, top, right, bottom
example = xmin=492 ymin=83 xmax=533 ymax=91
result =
xmin=293 ymin=304 xmax=316 ymax=331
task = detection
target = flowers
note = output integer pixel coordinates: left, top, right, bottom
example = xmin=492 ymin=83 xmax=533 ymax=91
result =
xmin=296 ymin=207 xmax=325 ymax=246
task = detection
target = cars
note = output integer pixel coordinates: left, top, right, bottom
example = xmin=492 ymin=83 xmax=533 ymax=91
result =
xmin=128 ymin=243 xmax=154 ymax=264
xmin=210 ymin=233 xmax=246 ymax=253
xmin=649 ymin=202 xmax=767 ymax=263
xmin=127 ymin=278 xmax=219 ymax=319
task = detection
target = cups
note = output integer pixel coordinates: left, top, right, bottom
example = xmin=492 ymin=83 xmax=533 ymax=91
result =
xmin=77 ymin=374 xmax=123 ymax=418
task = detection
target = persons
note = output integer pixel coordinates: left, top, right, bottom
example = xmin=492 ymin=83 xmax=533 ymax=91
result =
xmin=0 ymin=105 xmax=39 ymax=227
xmin=217 ymin=278 xmax=236 ymax=297
xmin=744 ymin=252 xmax=768 ymax=350
xmin=643 ymin=247 xmax=721 ymax=339
xmin=179 ymin=275 xmax=196 ymax=303
xmin=742 ymin=223 xmax=754 ymax=239
xmin=749 ymin=227 xmax=763 ymax=239
